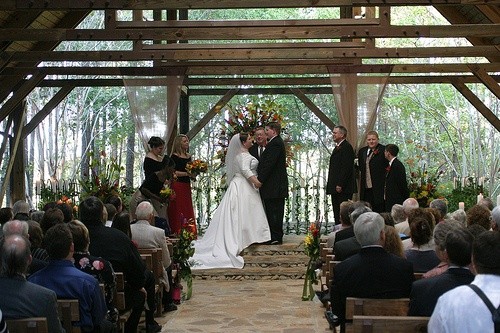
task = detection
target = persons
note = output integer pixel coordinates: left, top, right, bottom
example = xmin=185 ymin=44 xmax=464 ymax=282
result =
xmin=0 ymin=195 xmax=177 ymax=333
xmin=384 ymin=144 xmax=410 ymax=212
xmin=316 ymin=198 xmax=500 ymax=333
xmin=257 ymin=122 xmax=288 ymax=245
xmin=189 ymin=133 xmax=271 ymax=270
xmin=249 ymin=127 xmax=268 ymax=160
xmin=144 ymin=136 xmax=171 ymax=232
xmin=326 ymin=126 xmax=357 ymax=225
xmin=168 ymin=134 xmax=198 ymax=240
xmin=357 ymin=131 xmax=389 ymax=213
xmin=129 ymin=155 xmax=175 ymax=221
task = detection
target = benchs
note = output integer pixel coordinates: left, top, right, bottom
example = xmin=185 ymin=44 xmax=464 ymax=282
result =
xmin=318 ymin=240 xmax=426 ymax=333
xmin=6 ymin=238 xmax=180 ymax=333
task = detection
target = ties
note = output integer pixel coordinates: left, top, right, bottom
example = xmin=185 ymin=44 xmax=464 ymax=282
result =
xmin=260 ymin=145 xmax=263 ymax=155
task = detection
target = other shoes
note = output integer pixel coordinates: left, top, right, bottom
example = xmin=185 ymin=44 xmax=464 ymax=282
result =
xmin=146 ymin=323 xmax=163 ymax=333
xmin=164 ymin=304 xmax=177 ymax=312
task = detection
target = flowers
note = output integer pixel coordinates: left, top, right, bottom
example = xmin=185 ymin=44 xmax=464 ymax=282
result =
xmin=78 ymin=257 xmax=102 ymax=272
xmin=40 ymin=151 xmax=124 ymax=221
xmin=186 ymin=160 xmax=208 ymax=176
xmin=211 ymin=98 xmax=306 ymax=183
xmin=408 ymin=160 xmax=451 ymax=206
xmin=160 ymin=187 xmax=175 ymax=204
xmin=387 ymin=166 xmax=391 ymax=171
xmin=180 ymin=218 xmax=199 ymax=239
xmin=302 ymin=222 xmax=319 ymax=256
xmin=374 ymin=150 xmax=380 ymax=155
